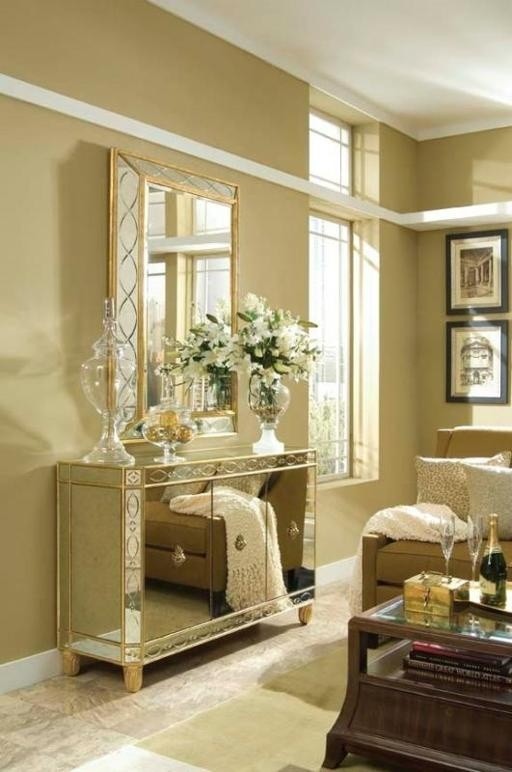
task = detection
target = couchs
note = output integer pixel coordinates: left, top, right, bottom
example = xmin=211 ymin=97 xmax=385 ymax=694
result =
xmin=363 ymin=426 xmax=512 ymax=615
xmin=145 ymin=453 xmax=308 ymax=619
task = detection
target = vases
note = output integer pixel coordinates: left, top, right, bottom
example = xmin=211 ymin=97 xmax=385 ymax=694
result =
xmin=247 ymin=367 xmax=288 ymax=455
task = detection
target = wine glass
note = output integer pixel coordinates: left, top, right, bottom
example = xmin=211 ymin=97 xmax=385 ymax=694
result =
xmin=465 ymin=514 xmax=484 ymax=584
xmin=438 ymin=516 xmax=458 ymax=577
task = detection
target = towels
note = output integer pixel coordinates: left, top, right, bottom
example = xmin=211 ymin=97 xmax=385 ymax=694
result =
xmin=171 ymin=484 xmax=287 ymax=611
xmin=342 ymin=503 xmax=478 ymax=617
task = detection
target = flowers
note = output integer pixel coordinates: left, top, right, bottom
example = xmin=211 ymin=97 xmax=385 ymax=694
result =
xmin=238 ymin=292 xmax=314 ymax=420
xmin=161 ymin=313 xmax=234 ymax=407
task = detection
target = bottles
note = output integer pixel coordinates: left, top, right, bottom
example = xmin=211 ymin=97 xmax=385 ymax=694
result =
xmin=478 ymin=513 xmax=507 ymax=606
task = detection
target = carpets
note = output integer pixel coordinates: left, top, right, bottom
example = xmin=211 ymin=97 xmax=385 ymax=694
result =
xmin=65 ymin=640 xmax=396 ymax=771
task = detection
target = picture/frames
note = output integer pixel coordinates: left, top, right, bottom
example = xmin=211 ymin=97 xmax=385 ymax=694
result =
xmin=445 ymin=229 xmax=505 ymax=315
xmin=445 ymin=320 xmax=508 ymax=404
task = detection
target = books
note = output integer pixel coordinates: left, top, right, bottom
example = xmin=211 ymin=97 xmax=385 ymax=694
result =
xmin=404 ymin=659 xmax=511 ymax=685
xmin=408 ymin=648 xmax=512 ymax=676
xmin=411 ymin=638 xmax=510 ymax=665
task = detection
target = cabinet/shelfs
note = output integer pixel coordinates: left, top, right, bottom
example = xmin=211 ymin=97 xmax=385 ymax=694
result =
xmin=55 ymin=448 xmax=323 ymax=690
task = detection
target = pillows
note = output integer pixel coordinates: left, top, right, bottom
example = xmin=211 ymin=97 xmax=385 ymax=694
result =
xmin=159 ymin=462 xmax=217 ymax=503
xmin=416 ymin=450 xmax=512 ymax=528
xmin=202 ymin=456 xmax=277 ymax=497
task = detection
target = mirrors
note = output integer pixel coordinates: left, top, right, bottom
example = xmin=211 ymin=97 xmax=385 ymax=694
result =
xmin=107 ymin=147 xmax=240 ymax=447
